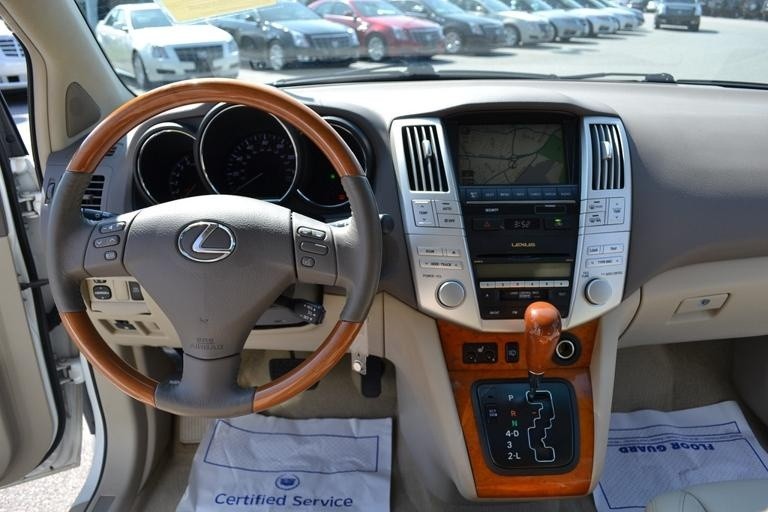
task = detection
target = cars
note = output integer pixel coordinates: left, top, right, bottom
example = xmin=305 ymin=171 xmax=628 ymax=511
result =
xmin=306 ymin=1 xmax=448 ymax=60
xmin=208 ymin=1 xmax=360 ymax=69
xmin=653 ymin=1 xmax=703 ymax=32
xmin=470 ymin=1 xmax=551 ymax=49
xmin=521 ymin=0 xmax=644 ymax=42
xmin=392 ymin=1 xmax=508 ymax=52
xmin=94 ymin=5 xmax=242 ymax=84
